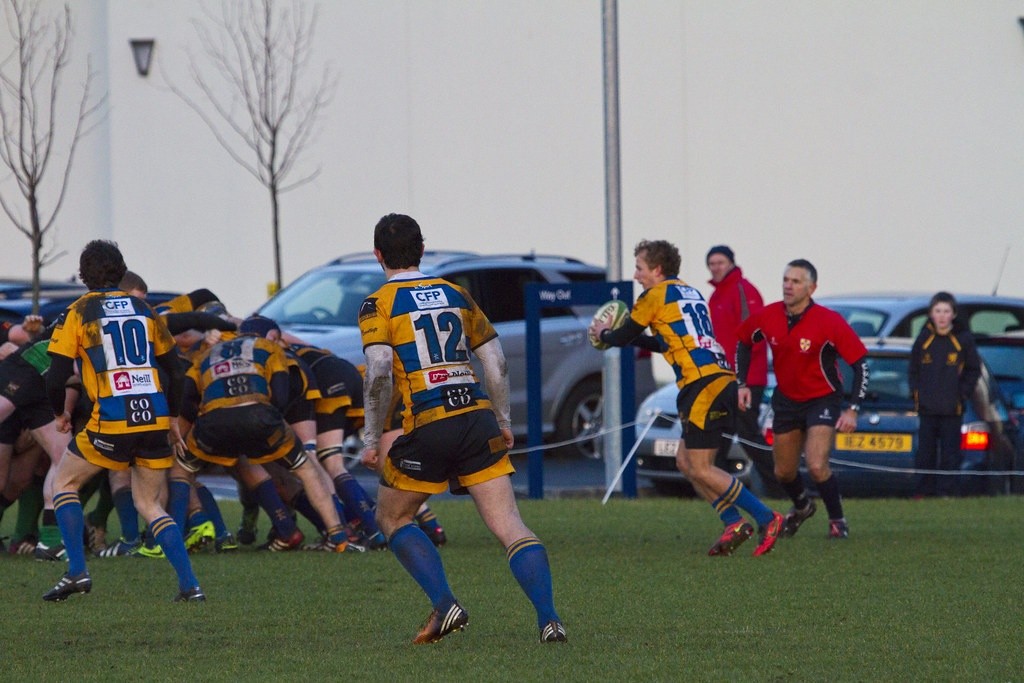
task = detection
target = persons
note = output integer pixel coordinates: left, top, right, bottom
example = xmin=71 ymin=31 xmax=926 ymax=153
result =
xmin=361 ymin=212 xmax=567 ymax=646
xmin=736 ymin=259 xmax=871 ymax=541
xmin=0 ymin=237 xmax=448 ymax=603
xmin=909 ymin=292 xmax=981 ymax=500
xmin=587 ymin=240 xmax=784 ymax=558
xmin=706 ymin=246 xmax=787 ymax=501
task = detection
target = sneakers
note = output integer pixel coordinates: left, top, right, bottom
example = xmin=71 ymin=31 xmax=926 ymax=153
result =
xmin=538 ymin=621 xmax=567 ymax=643
xmin=780 ymin=498 xmax=816 ymax=538
xmin=238 ymin=520 xmax=445 ymax=556
xmin=708 ymin=516 xmax=753 ymax=557
xmin=0 ymin=512 xmax=238 ymax=561
xmin=171 ymin=586 xmax=206 ymax=605
xmin=753 ymin=511 xmax=783 ymax=558
xmin=411 ymin=601 xmax=468 ymax=646
xmin=44 ymin=572 xmax=93 ymax=605
xmin=828 ymin=520 xmax=849 ymax=542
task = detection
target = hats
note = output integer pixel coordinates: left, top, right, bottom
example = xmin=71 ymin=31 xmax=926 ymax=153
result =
xmin=707 ymin=245 xmax=733 ymax=263
xmin=239 ymin=313 xmax=281 ymax=339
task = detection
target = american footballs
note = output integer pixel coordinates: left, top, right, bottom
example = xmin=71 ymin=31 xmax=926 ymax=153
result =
xmin=587 ymin=301 xmax=631 ymax=350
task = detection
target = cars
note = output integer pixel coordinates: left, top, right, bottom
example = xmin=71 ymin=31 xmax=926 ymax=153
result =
xmin=633 ymin=287 xmax=1024 ymax=503
xmin=244 ymin=244 xmax=640 ymax=475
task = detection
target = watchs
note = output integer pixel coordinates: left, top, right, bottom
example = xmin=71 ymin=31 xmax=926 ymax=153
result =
xmin=849 ymin=404 xmax=860 ymax=411
